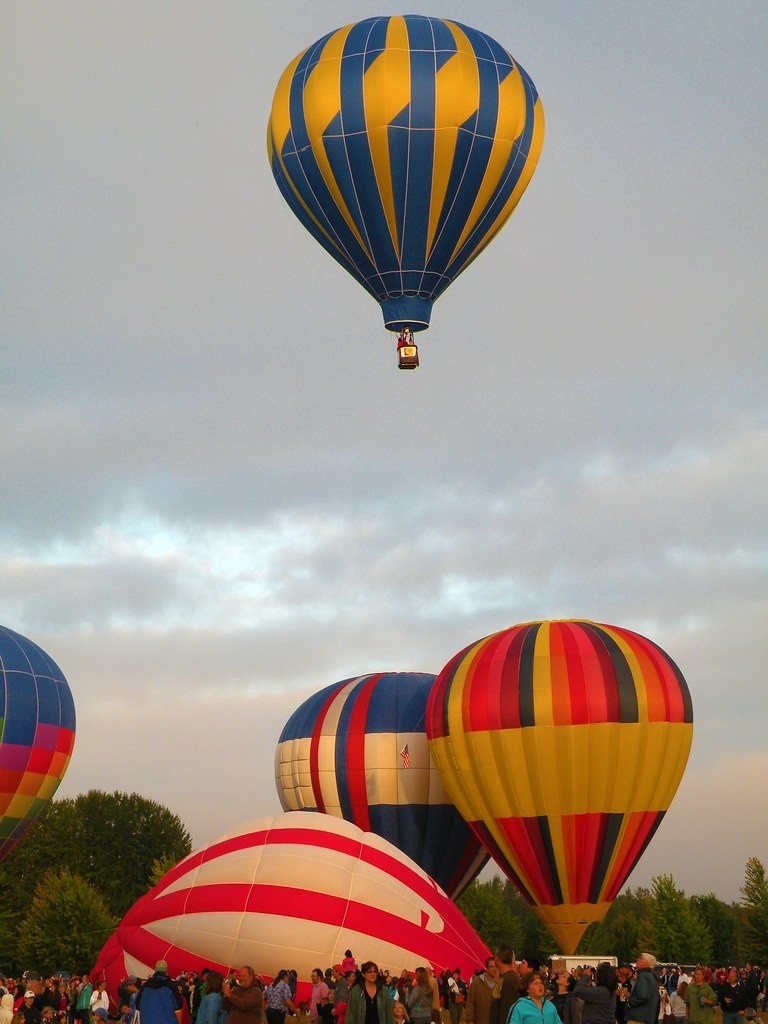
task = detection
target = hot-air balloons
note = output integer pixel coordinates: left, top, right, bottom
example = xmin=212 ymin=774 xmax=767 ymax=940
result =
xmin=424 ymin=618 xmax=695 ymax=954
xmin=274 ymin=670 xmax=490 ymax=904
xmin=89 ymin=808 xmax=502 ymax=1015
xmin=265 ymin=13 xmax=547 ymax=371
xmin=1 ymin=624 xmax=76 ymax=862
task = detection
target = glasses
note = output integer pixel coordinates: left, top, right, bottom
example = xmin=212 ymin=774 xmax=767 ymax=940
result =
xmin=366 ymin=970 xmax=378 ymax=973
xmin=311 ymin=975 xmax=318 ymax=978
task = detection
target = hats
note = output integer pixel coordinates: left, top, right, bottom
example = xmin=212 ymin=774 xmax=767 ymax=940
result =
xmin=24 ymin=991 xmax=35 ymax=998
xmin=155 ymin=960 xmax=167 ymax=971
xmin=615 ymin=963 xmax=633 ymax=969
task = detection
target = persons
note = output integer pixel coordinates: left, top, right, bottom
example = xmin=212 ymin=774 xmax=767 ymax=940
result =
xmin=0 ymin=949 xmax=768 ymax=1024
xmin=397 ymin=334 xmax=415 ymax=349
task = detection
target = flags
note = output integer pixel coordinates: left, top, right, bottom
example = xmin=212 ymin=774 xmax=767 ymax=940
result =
xmin=400 ymin=745 xmax=410 ymax=769
